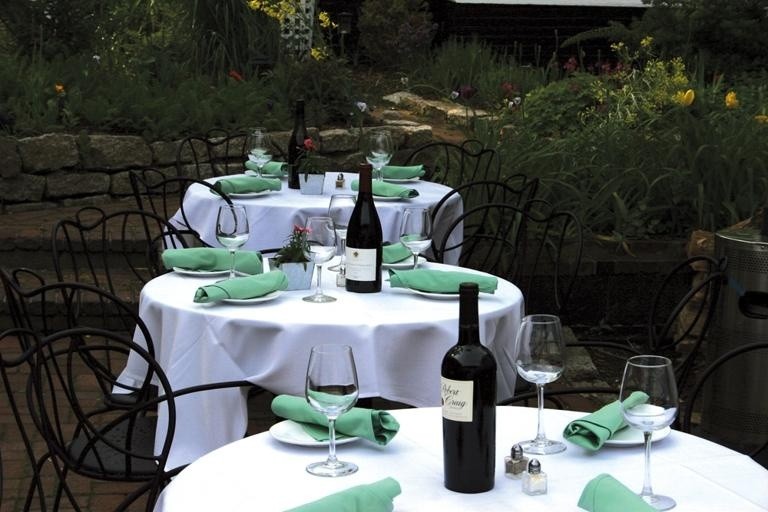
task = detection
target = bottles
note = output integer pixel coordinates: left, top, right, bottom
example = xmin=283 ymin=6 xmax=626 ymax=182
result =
xmin=441 ymin=283 xmax=548 ymax=495
xmin=344 ymin=164 xmax=384 ymax=293
xmin=287 ymin=99 xmax=312 ymax=189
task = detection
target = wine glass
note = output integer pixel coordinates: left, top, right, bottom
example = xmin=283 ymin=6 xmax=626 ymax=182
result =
xmin=305 ymin=345 xmax=359 ymax=477
xmin=398 ymin=206 xmax=433 ymax=270
xmin=301 ymin=216 xmax=338 ymax=302
xmin=218 ymin=205 xmax=251 ymax=280
xmin=619 ymin=355 xmax=680 ymax=511
xmin=248 ymin=134 xmax=273 ymax=178
xmin=328 ymin=194 xmax=357 ymax=272
xmin=514 ymin=313 xmax=566 ymax=455
xmin=366 ymin=134 xmax=390 ymax=181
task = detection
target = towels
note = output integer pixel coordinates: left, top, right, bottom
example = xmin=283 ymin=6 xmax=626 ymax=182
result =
xmin=568 ymin=391 xmax=649 ymax=451
xmin=577 ymin=473 xmax=658 ymax=512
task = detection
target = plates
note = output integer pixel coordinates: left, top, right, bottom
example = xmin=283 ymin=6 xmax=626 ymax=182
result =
xmin=228 ymin=189 xmax=271 ymax=198
xmin=268 ymin=420 xmax=361 ymax=448
xmin=173 ymin=264 xmax=231 ymax=279
xmin=222 ymin=290 xmax=283 ymax=302
xmin=173 ymin=169 xmax=461 ymax=305
xmin=372 ymin=177 xmax=420 ymax=184
xmin=245 ymin=170 xmax=288 ymax=178
xmin=381 ymin=255 xmax=427 ymax=269
xmin=406 ymin=285 xmax=460 ymax=302
xmin=371 ymin=194 xmax=411 ymax=201
xmin=603 ymin=425 xmax=673 ymax=449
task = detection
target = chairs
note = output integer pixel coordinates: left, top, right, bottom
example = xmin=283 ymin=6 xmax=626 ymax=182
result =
xmin=684 ymin=341 xmax=768 ymax=458
xmin=497 ymin=257 xmax=720 ymax=431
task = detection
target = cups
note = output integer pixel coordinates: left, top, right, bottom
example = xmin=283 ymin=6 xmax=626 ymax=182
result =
xmin=245 ymin=126 xmax=265 ymax=159
xmin=374 ymin=129 xmax=394 ymax=164
